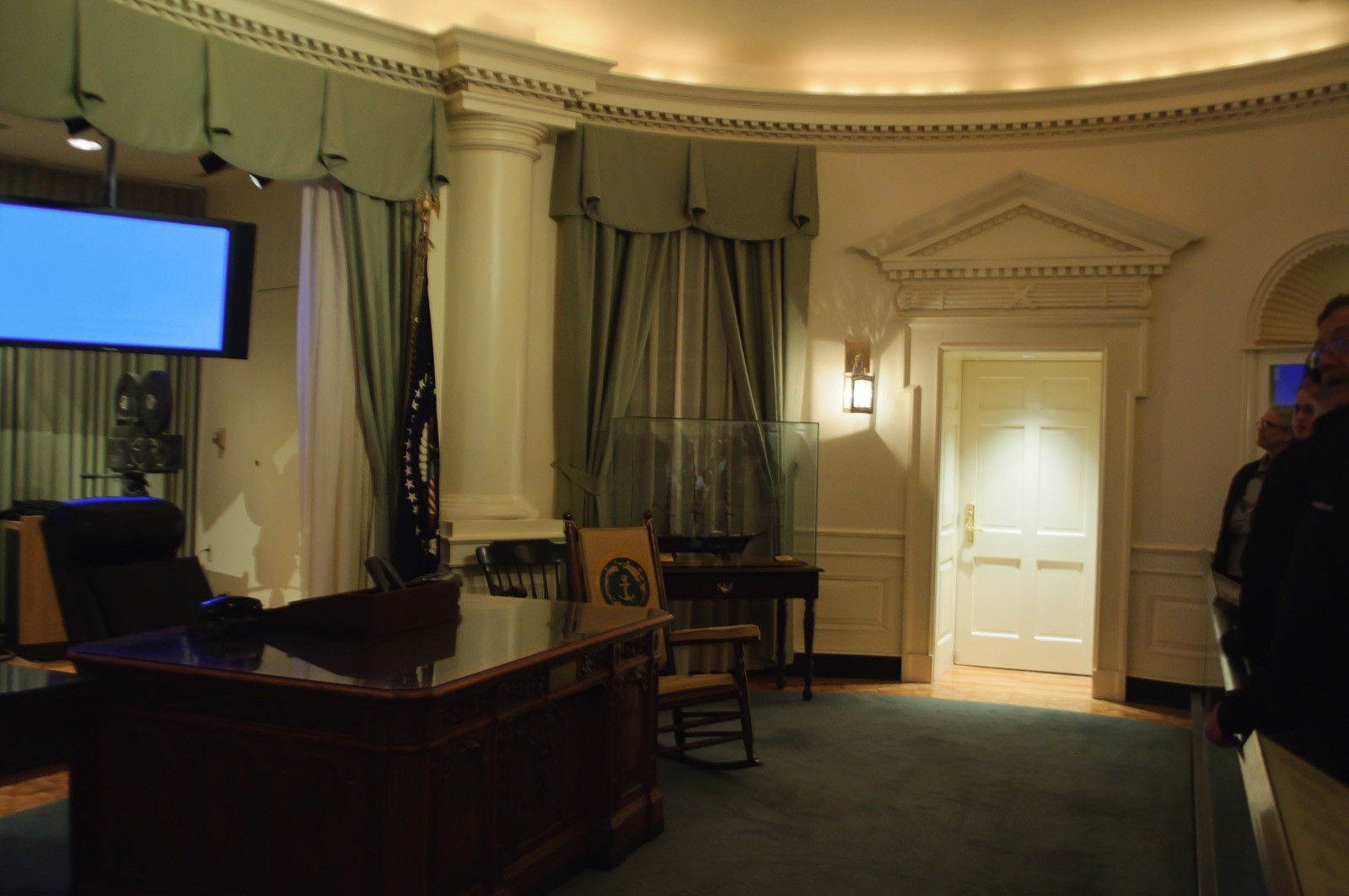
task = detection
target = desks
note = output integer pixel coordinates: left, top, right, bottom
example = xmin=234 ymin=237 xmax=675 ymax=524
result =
xmin=62 ymin=590 xmax=673 ymax=895
xmin=568 ymin=554 xmax=825 ymax=702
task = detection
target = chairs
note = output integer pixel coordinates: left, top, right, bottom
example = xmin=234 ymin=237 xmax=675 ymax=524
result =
xmin=38 ymin=494 xmax=214 ymax=644
xmin=563 ymin=509 xmax=764 ymax=770
xmin=476 ymin=539 xmax=571 ymax=603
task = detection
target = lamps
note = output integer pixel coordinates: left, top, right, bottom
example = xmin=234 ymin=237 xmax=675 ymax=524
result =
xmin=249 ymin=173 xmax=269 ymax=189
xmin=843 ymin=353 xmax=875 ymax=414
xmin=199 ymin=150 xmax=228 ymax=172
xmin=66 ymin=118 xmax=104 ymax=152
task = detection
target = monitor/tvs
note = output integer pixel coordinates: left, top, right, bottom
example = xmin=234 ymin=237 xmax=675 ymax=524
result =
xmin=1 ymin=198 xmax=259 ymax=359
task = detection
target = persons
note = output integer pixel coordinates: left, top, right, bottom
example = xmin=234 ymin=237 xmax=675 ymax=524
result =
xmin=1206 ymin=293 xmax=1348 ymax=790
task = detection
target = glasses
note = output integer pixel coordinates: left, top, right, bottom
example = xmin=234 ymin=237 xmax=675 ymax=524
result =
xmin=1255 ymin=418 xmax=1283 ymax=430
xmin=1304 ymin=324 xmax=1349 ymax=372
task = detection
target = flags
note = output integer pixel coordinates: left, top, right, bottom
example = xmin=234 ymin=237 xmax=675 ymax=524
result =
xmin=393 ymin=264 xmax=443 ymax=579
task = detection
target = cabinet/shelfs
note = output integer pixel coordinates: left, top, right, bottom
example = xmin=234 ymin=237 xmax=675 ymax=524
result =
xmin=2 ymin=515 xmax=71 ymax=659
